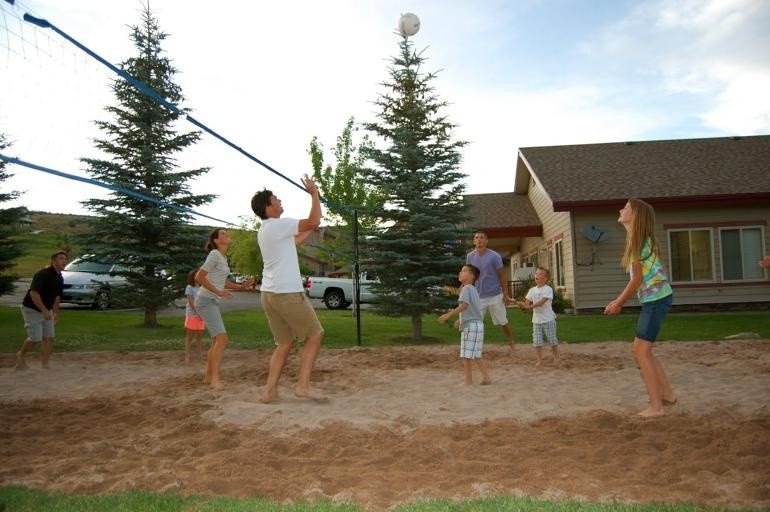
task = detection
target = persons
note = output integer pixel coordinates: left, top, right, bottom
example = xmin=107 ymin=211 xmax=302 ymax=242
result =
xmin=16 ymin=250 xmax=67 ymax=368
xmin=437 ymin=264 xmax=493 ymax=386
xmin=193 ymin=227 xmax=256 ymax=387
xmin=517 ymin=267 xmax=567 ymax=366
xmin=603 ymin=198 xmax=677 ymax=418
xmin=466 ymin=230 xmax=515 ymax=354
xmin=184 ymin=268 xmax=205 ymax=367
xmin=251 ymin=173 xmax=328 ymax=402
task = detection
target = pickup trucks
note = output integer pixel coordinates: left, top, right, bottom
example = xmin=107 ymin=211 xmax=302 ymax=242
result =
xmin=306 ymin=270 xmax=450 ymax=310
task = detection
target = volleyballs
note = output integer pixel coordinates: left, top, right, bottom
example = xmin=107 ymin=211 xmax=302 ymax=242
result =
xmin=399 ymin=13 xmax=420 ymax=35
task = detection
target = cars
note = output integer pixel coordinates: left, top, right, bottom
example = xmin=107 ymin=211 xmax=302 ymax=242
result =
xmin=227 ymin=271 xmax=262 ymax=287
xmin=56 ymin=250 xmax=170 ymax=310
